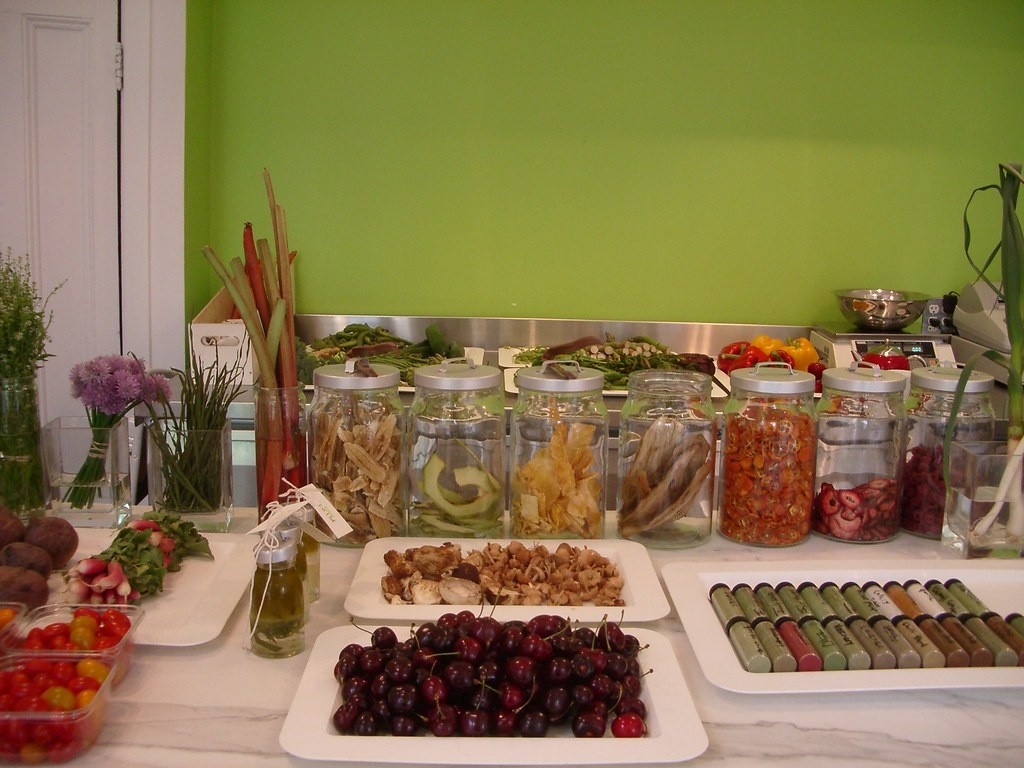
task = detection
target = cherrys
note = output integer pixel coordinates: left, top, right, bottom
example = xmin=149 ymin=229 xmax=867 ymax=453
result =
xmin=332 ymin=586 xmax=655 ymax=738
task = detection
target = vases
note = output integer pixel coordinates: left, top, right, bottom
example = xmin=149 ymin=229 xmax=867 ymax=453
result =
xmin=0 ymin=384 xmax=46 ymax=518
xmin=40 ymin=416 xmax=126 ymax=528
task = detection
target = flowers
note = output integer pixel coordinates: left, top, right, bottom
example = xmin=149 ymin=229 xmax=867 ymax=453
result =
xmin=69 ymin=353 xmax=169 ymax=427
xmin=0 ymin=246 xmax=70 ymax=376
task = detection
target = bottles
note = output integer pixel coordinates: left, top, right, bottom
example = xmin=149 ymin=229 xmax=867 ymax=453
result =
xmin=508 ymin=359 xmax=607 ymax=540
xmin=405 ymin=357 xmax=510 ymax=540
xmin=899 ymin=365 xmax=997 ymax=540
xmin=305 ymin=357 xmax=407 ymax=549
xmin=814 ymin=361 xmax=905 ymax=545
xmin=616 ymin=369 xmax=718 ymax=550
xmin=716 ymin=361 xmax=818 ymax=548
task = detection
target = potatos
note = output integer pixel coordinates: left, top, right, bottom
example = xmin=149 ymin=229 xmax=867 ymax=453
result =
xmin=0 ymin=505 xmax=79 ymax=610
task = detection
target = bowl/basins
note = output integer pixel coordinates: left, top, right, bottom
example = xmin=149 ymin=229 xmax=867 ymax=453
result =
xmin=830 ymin=288 xmax=932 ymax=331
xmin=0 ymin=653 xmax=118 ymax=765
xmin=1 ymin=601 xmax=146 ymax=692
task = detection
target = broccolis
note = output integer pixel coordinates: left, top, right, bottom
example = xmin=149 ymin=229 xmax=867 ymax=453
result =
xmin=291 ymin=332 xmax=348 ymax=385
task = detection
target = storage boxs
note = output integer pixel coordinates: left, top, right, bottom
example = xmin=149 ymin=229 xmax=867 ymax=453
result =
xmin=0 ymin=601 xmax=145 ymax=767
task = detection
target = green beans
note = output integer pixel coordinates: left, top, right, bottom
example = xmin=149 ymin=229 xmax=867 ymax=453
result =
xmin=344 ymin=323 xmax=412 ymax=346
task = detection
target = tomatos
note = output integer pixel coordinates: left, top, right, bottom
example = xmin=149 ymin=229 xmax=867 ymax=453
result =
xmin=0 ymin=605 xmax=135 ymax=762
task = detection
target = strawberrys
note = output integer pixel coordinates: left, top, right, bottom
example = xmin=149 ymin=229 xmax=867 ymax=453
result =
xmin=813 ymin=478 xmax=900 ymax=541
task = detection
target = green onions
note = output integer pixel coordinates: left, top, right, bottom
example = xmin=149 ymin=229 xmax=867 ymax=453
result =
xmin=940 ymin=159 xmax=1024 ymax=545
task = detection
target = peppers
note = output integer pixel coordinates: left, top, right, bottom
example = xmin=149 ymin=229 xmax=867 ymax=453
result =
xmin=403 ymin=323 xmax=466 ymax=357
xmin=716 ymin=334 xmax=830 ymax=394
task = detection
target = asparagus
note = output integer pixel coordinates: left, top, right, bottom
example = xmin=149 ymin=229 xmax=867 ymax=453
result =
xmin=578 ymin=332 xmax=669 ymax=359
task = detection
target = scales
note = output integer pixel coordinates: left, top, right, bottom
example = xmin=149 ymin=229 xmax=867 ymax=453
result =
xmin=809 ymin=320 xmax=958 ymax=368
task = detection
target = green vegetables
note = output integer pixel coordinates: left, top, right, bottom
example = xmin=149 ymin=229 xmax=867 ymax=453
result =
xmin=365 ymin=352 xmax=447 ymax=382
xmin=512 ymin=344 xmax=702 ymax=391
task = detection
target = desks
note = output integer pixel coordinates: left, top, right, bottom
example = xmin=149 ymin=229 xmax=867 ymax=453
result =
xmin=20 ymin=495 xmax=1024 ymax=768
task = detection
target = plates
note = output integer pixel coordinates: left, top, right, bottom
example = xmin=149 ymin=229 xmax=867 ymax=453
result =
xmin=279 ymin=624 xmax=710 ymax=765
xmin=48 ymin=541 xmax=258 ymax=648
xmin=344 ymin=536 xmax=672 ymax=623
xmin=662 ymin=558 xmax=1023 ymax=694
